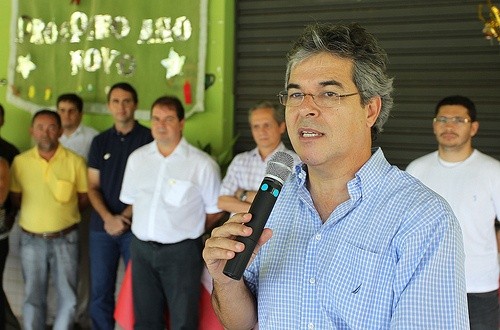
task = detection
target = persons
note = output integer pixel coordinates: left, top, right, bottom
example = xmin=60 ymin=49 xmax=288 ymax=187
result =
xmin=0 ymin=92 xmax=100 ymax=330
xmin=405 ymin=96 xmax=500 ymax=330
xmin=202 ymin=23 xmax=470 ymax=330
xmin=83 ymin=82 xmax=154 ymax=330
xmin=216 ymin=97 xmax=302 ymax=217
xmin=120 ymin=96 xmax=223 ymax=330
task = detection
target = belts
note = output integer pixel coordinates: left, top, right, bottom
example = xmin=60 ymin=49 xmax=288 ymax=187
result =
xmin=19 ymin=222 xmax=80 ymax=239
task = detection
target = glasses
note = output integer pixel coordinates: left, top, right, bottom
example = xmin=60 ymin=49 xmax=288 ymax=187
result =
xmin=277 ymin=88 xmax=359 ymax=108
xmin=433 ymin=116 xmax=472 ymax=127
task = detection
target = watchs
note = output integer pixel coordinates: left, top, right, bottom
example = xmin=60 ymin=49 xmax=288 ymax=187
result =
xmin=238 ymin=190 xmax=248 ymax=203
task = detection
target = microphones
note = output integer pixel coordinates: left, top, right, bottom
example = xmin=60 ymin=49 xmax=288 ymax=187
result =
xmin=223 ymin=151 xmax=294 ymax=280
